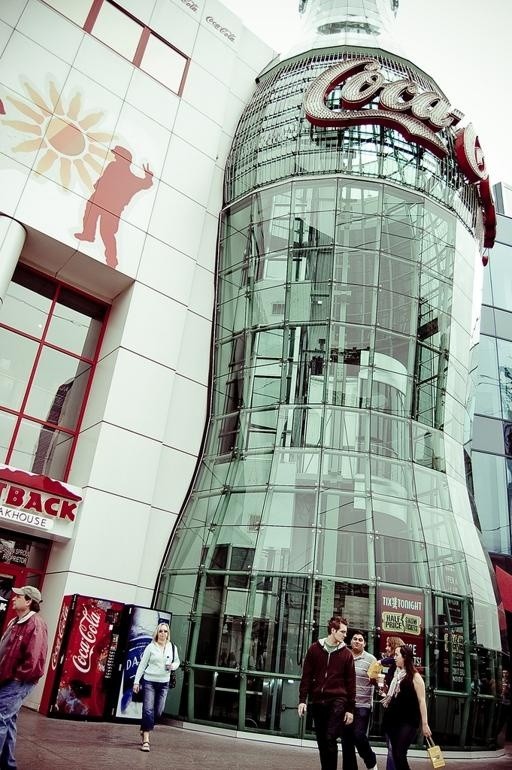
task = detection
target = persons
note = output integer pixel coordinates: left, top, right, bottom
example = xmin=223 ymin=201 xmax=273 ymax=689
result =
xmin=296 ymin=614 xmax=358 ymax=769
xmin=1 ymin=584 xmax=49 ymax=770
xmin=74 ymin=143 xmax=155 ymax=271
xmin=341 ymin=625 xmax=381 ymax=769
xmin=367 ymin=633 xmax=408 ymax=769
xmin=131 ymin=621 xmax=183 ymax=754
xmin=380 ymin=644 xmax=432 ymax=769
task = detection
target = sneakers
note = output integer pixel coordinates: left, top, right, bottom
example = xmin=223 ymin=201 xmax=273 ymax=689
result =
xmin=368 ymin=763 xmax=378 ymax=770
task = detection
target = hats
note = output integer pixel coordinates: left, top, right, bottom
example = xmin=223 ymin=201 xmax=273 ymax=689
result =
xmin=12 ymin=585 xmax=42 ymax=603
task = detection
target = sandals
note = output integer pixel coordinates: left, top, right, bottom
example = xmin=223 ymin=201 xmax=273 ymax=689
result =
xmin=139 ymin=730 xmax=151 ymax=752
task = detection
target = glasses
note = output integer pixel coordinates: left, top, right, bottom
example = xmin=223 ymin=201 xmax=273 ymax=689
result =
xmin=158 ymin=630 xmax=168 ymax=633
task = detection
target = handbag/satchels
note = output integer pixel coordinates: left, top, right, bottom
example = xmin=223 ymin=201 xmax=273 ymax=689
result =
xmin=427 ymin=745 xmax=445 ymax=769
xmin=169 ymin=671 xmax=176 ymax=689
